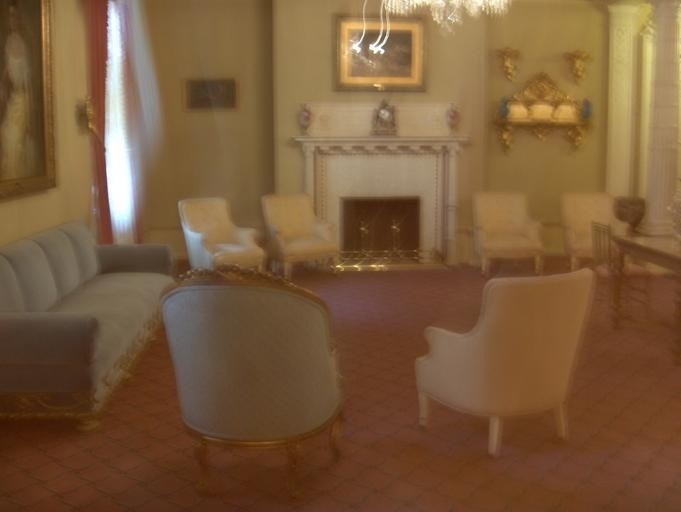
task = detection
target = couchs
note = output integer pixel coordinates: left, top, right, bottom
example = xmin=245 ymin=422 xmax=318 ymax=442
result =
xmin=0 ymin=222 xmax=176 ymax=432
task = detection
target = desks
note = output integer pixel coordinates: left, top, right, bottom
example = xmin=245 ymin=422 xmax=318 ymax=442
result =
xmin=610 ymin=234 xmax=681 ymax=365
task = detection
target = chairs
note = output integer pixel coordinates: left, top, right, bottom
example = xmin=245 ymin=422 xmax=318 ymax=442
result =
xmin=471 ymin=191 xmax=652 ymax=312
xmin=415 ymin=268 xmax=593 ymax=457
xmin=177 ymin=192 xmax=339 ymax=279
xmin=158 ymin=262 xmax=347 ymax=502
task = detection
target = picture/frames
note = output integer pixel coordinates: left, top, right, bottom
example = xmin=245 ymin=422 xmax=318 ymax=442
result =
xmin=0 ymin=0 xmax=58 ymax=203
xmin=332 ymin=14 xmax=429 ymax=92
